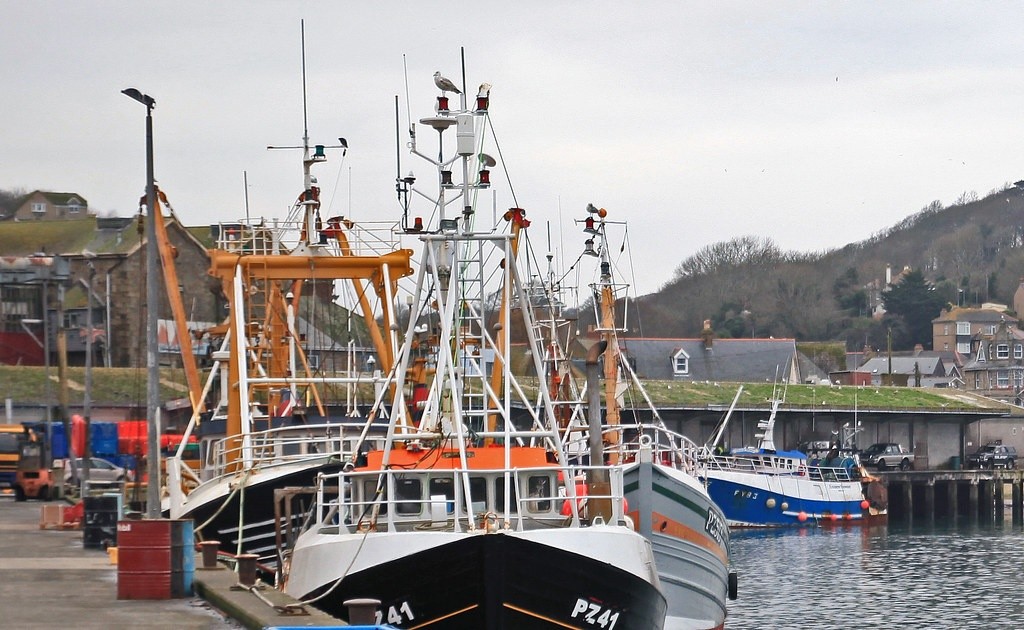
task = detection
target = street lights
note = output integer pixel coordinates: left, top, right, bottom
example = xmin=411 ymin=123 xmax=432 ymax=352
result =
xmin=119 ymin=87 xmax=161 ymax=521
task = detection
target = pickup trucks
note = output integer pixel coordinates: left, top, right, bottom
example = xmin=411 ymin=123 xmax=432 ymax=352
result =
xmin=860 ymin=443 xmax=914 ymax=472
xmin=965 ymin=441 xmax=1019 ymax=471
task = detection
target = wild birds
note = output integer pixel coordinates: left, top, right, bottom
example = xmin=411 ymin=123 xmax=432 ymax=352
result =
xmin=338 ymin=138 xmax=348 ymax=148
xmin=432 ymin=70 xmax=463 ymax=98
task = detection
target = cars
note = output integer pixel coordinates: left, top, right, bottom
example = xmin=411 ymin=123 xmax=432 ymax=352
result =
xmin=63 ymin=459 xmax=135 ymax=487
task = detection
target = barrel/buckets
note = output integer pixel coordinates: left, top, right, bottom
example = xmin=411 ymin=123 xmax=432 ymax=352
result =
xmin=116 ymin=520 xmax=172 ymax=599
xmin=171 ymin=519 xmax=195 ymax=597
xmin=82 ymin=495 xmax=117 ymax=550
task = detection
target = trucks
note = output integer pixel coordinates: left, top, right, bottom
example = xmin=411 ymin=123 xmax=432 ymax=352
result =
xmin=0 ymin=424 xmax=36 ymax=489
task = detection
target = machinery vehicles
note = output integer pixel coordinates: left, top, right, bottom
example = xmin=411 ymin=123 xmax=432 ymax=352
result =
xmin=13 ymin=424 xmax=53 ymax=502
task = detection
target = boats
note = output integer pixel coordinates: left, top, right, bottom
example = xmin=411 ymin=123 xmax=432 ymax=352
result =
xmin=161 ymin=17 xmax=873 ymax=630
